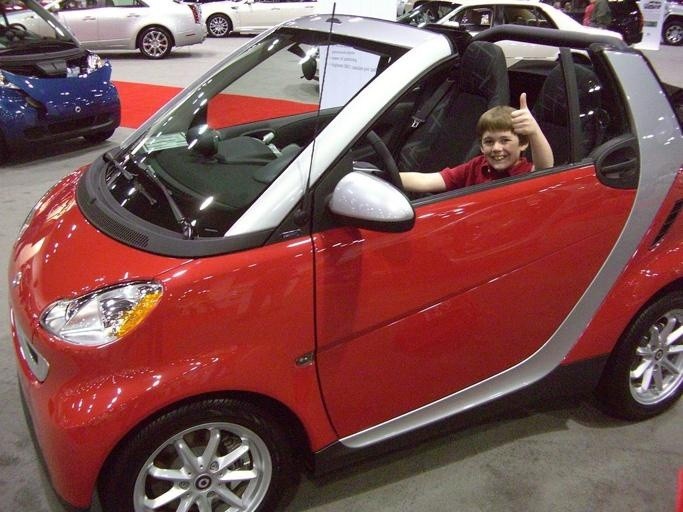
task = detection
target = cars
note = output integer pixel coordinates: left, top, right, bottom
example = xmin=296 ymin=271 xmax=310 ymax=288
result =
xmin=662 ymin=0 xmax=683 ymax=47
xmin=7 ymin=13 xmax=683 ymax=511
xmin=1 ymin=0 xmax=207 ymax=60
xmin=199 ymin=0 xmax=318 ymax=38
xmin=0 ymin=0 xmax=122 ymax=166
xmin=417 ymin=1 xmax=624 ymax=70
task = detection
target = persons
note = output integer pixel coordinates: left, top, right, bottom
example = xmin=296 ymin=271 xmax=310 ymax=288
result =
xmin=552 ymin=1 xmax=563 ymax=12
xmin=582 ymin=0 xmax=598 ymax=27
xmin=564 ymin=2 xmax=574 ymax=13
xmin=396 ymin=91 xmax=555 ymax=199
xmin=589 ymin=0 xmax=612 ymax=30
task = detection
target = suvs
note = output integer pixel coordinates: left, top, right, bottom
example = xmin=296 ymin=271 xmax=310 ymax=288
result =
xmin=539 ymin=1 xmax=644 ymax=46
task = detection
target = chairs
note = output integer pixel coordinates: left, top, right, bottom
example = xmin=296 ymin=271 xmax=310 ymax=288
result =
xmin=413 ymin=40 xmax=510 ymax=198
xmin=524 ymin=60 xmax=603 ymax=169
